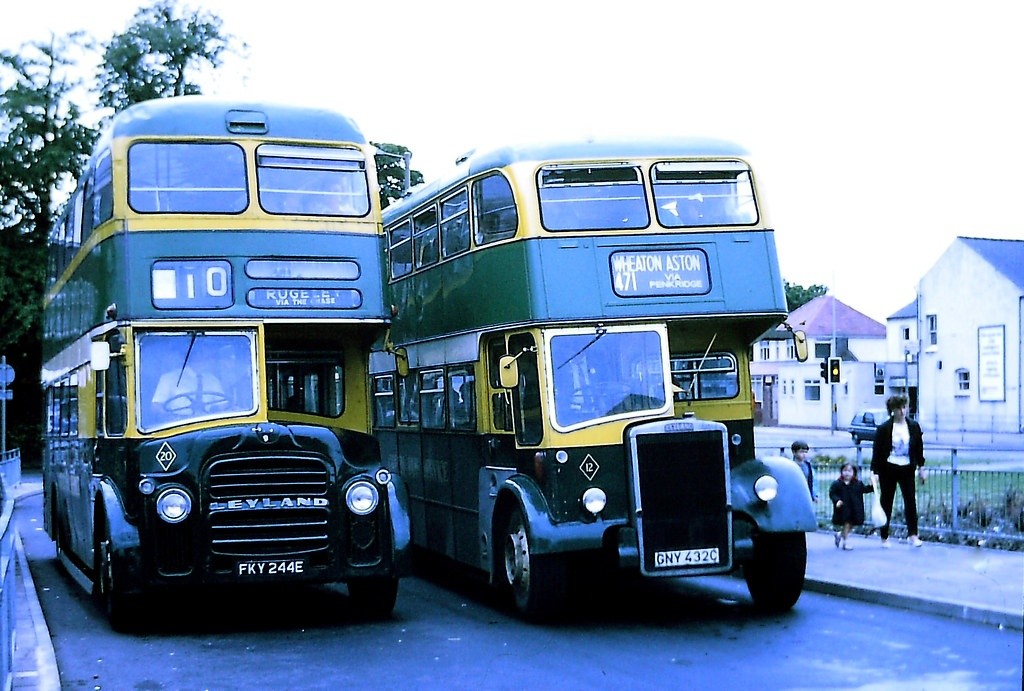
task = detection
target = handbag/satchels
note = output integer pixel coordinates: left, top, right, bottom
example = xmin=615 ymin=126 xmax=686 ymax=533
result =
xmin=871 ymin=476 xmax=887 ymax=527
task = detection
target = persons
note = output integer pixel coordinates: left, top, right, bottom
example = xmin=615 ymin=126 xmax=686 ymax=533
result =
xmin=789 ymin=440 xmax=818 ymax=508
xmin=537 ymin=180 xmax=749 ymax=230
xmin=151 ymin=344 xmax=232 ymax=419
xmin=380 ymin=372 xmax=479 ymax=427
xmin=553 ymin=339 xmax=609 ymax=431
xmin=871 ymin=394 xmax=929 ymax=549
xmin=828 ymin=463 xmax=873 ymax=551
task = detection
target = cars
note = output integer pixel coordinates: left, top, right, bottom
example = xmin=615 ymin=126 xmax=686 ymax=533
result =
xmin=848 ymin=408 xmax=893 ymax=444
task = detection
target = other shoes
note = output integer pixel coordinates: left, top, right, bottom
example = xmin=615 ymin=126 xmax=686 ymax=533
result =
xmin=834 ymin=531 xmax=842 ymax=547
xmin=842 ymin=544 xmax=853 ymax=550
xmin=881 ymin=541 xmax=891 ymax=548
xmin=907 ymin=536 xmax=922 ymax=546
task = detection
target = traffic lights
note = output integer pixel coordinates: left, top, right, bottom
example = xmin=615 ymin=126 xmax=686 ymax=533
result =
xmin=828 ymin=357 xmax=841 ymax=385
xmin=820 ymin=357 xmax=829 ymax=384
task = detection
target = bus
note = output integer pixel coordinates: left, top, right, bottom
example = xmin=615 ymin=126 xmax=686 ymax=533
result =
xmin=368 ymin=135 xmax=819 ymax=624
xmin=40 ymin=93 xmax=417 ymax=635
